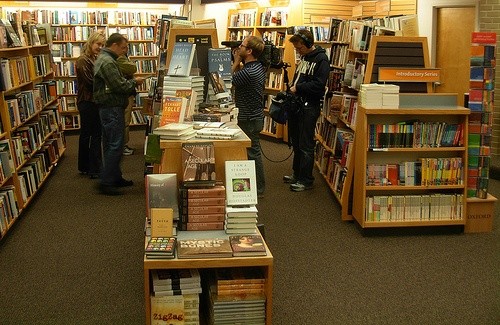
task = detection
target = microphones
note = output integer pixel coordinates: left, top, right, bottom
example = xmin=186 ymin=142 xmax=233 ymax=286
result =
xmin=221 ymin=41 xmax=240 ymax=47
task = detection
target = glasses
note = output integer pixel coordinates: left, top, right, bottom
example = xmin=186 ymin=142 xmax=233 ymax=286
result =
xmin=240 ymin=43 xmax=247 ymax=49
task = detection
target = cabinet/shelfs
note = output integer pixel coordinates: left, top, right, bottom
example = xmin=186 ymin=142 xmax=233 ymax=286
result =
xmin=0 ymin=8 xmax=470 ymax=325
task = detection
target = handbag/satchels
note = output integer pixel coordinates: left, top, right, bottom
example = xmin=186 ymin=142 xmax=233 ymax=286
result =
xmin=268 ymin=92 xmax=303 ymax=125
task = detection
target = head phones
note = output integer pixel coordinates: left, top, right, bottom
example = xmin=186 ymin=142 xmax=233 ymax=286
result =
xmin=295 ymin=32 xmax=313 ymax=48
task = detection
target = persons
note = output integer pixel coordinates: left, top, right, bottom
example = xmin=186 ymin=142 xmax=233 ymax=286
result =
xmin=231 ymin=36 xmax=266 ymax=198
xmin=93 ymin=32 xmax=143 ymax=196
xmin=75 ymin=31 xmax=107 ymax=180
xmin=283 ymin=29 xmax=330 ymax=192
xmin=115 ymin=42 xmax=137 ymax=141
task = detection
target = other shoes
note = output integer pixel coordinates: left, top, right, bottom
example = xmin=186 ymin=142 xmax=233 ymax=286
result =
xmin=290 ymin=183 xmax=314 ymax=192
xmin=122 ymin=146 xmax=136 ymax=156
xmin=283 ymin=175 xmax=299 ymax=183
xmin=96 ymin=182 xmax=124 ymax=196
xmin=80 ymin=168 xmax=98 ymax=178
xmin=112 ymin=177 xmax=133 ymax=187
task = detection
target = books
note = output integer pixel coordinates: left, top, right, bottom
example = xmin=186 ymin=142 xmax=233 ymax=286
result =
xmin=150 ymin=268 xmax=266 ymax=325
xmin=144 ymin=234 xmax=268 ymax=259
xmin=0 ymin=7 xmax=497 ymax=237
